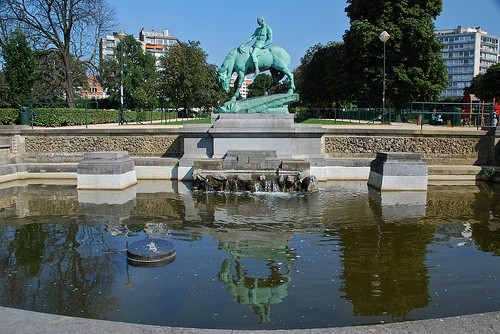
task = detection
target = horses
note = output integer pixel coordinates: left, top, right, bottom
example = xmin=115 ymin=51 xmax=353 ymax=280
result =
xmin=216 ymin=46 xmax=296 ymax=102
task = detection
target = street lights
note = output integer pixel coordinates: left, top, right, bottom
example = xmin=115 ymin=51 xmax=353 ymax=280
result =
xmin=114 ymin=30 xmax=129 ymax=124
xmin=378 ymin=31 xmax=390 ymax=123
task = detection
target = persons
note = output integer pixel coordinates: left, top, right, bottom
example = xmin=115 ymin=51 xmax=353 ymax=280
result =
xmin=243 ymin=278 xmax=272 ymax=325
xmin=240 ymin=16 xmax=273 ymax=77
